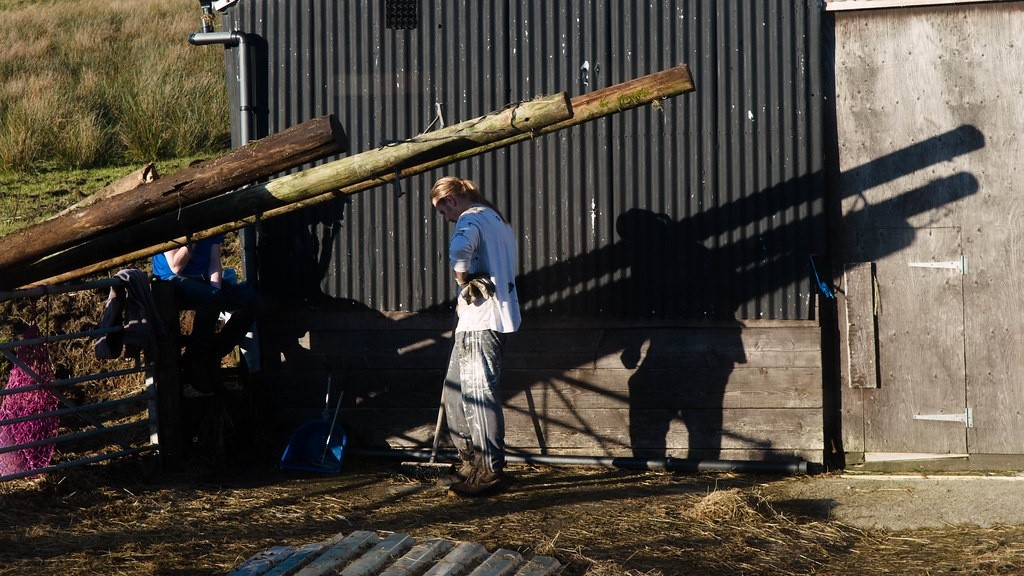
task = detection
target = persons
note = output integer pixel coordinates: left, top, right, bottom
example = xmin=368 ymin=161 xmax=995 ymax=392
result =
xmin=152 ymin=234 xmax=257 ymax=394
xmin=430 ymin=176 xmax=522 ymax=493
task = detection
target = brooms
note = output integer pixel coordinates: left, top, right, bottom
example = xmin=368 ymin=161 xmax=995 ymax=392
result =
xmin=395 ymin=377 xmax=457 ymax=478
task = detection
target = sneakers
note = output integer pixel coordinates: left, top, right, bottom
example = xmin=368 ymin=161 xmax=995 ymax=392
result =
xmin=448 ymin=467 xmax=506 ymax=497
xmin=437 ymin=455 xmax=476 ymax=489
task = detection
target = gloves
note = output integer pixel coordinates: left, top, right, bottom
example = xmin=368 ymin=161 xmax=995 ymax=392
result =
xmin=455 ymin=272 xmax=496 ymax=305
